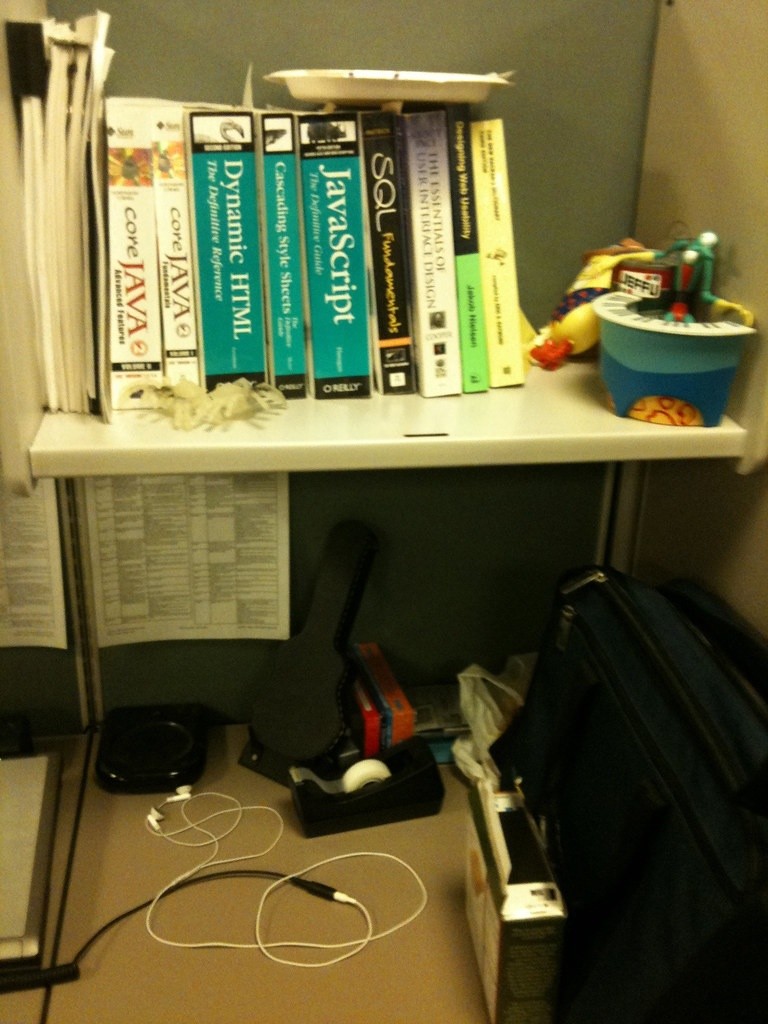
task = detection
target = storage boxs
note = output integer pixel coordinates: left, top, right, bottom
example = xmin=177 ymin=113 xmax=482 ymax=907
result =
xmin=463 ymin=774 xmax=570 ymax=1023
xmin=349 ymin=640 xmax=417 ymax=757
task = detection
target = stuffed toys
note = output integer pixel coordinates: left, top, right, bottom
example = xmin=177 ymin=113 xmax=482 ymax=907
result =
xmin=530 ymin=238 xmax=688 ymax=371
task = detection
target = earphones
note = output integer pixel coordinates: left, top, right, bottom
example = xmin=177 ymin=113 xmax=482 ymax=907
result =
xmin=165 ymin=786 xmax=193 ymax=804
xmin=145 ymin=806 xmax=164 ymax=832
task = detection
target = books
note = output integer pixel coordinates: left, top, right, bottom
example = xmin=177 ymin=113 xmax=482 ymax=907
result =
xmin=19 ymin=10 xmax=523 ymax=424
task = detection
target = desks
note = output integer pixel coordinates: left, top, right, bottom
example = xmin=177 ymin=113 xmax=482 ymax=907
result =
xmin=24 ymin=359 xmax=748 ymax=737
